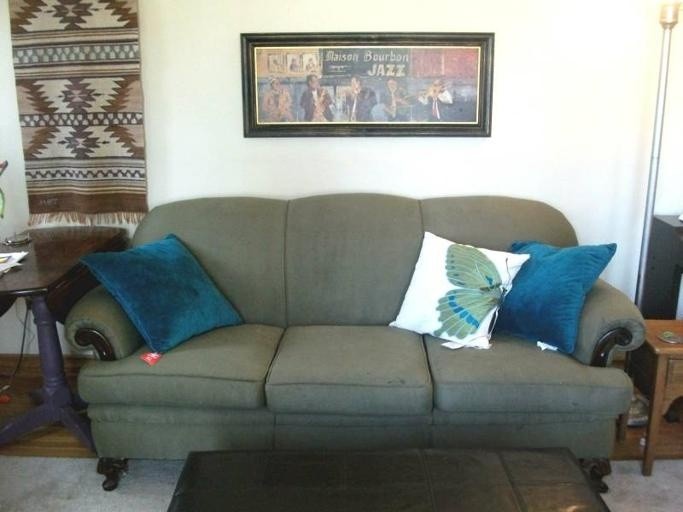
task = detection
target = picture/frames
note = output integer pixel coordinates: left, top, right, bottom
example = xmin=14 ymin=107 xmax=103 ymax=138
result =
xmin=241 ymin=33 xmax=494 ymax=136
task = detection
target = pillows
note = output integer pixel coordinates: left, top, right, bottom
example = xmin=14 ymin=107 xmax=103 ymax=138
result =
xmin=391 ymin=230 xmax=618 ymax=354
xmin=76 ymin=234 xmax=244 ymax=353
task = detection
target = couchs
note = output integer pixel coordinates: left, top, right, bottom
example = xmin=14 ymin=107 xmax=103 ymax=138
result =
xmin=66 ymin=194 xmax=646 ymax=493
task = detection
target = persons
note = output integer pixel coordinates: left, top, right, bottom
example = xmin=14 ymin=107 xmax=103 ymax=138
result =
xmin=261 ymin=59 xmax=454 ymax=122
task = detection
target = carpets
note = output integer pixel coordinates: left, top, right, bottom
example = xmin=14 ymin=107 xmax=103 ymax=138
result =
xmin=0 ymin=456 xmax=683 ymax=511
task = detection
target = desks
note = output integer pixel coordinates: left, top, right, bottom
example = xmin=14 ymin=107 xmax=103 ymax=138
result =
xmin=616 ymin=319 xmax=683 ymax=475
xmin=1 ymin=226 xmax=126 ymax=457
